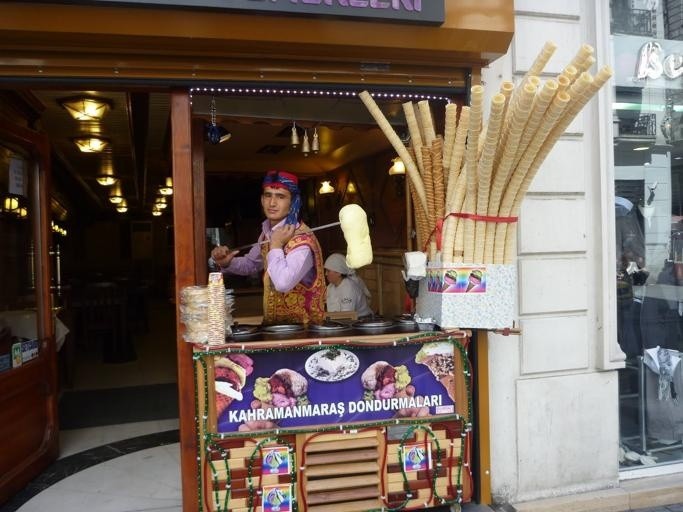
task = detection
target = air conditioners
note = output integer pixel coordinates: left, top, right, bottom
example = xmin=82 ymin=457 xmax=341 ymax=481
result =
xmin=129 ymin=221 xmax=154 ymax=289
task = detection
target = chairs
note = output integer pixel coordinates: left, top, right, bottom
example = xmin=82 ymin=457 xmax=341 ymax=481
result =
xmin=19 ymin=262 xmax=147 ymax=363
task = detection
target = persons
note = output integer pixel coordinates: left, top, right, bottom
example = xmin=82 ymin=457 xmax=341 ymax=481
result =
xmin=234 ymin=400 xmax=283 ymax=432
xmin=323 ymin=253 xmax=374 ymax=320
xmin=611 ymin=191 xmax=650 ymax=407
xmin=209 ymin=169 xmax=325 ymax=320
xmin=636 ymin=217 xmax=683 ymax=448
xmin=393 ymin=384 xmax=435 ymax=417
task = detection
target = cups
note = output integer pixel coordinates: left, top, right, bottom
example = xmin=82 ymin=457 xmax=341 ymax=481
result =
xmin=206 ymin=272 xmax=225 ymax=346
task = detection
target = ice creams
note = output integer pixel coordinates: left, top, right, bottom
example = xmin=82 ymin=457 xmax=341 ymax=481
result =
xmin=216 ymin=354 xmax=257 ymax=420
xmin=465 ymin=270 xmax=483 ymax=293
xmin=358 ymin=362 xmax=430 ymax=418
xmin=431 ymin=275 xmax=440 ymax=291
xmin=414 ymin=343 xmax=456 ymax=405
xmin=426 ymin=275 xmax=434 ymax=291
xmin=238 ymin=366 xmax=313 ymax=429
xmin=438 ymin=270 xmax=458 ymax=292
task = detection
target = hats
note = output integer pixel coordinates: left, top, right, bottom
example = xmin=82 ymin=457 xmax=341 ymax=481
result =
xmin=263 ymin=170 xmax=301 ymax=226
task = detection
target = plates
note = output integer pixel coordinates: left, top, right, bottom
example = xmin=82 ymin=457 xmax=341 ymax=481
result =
xmin=304 ymin=348 xmax=360 ymax=382
